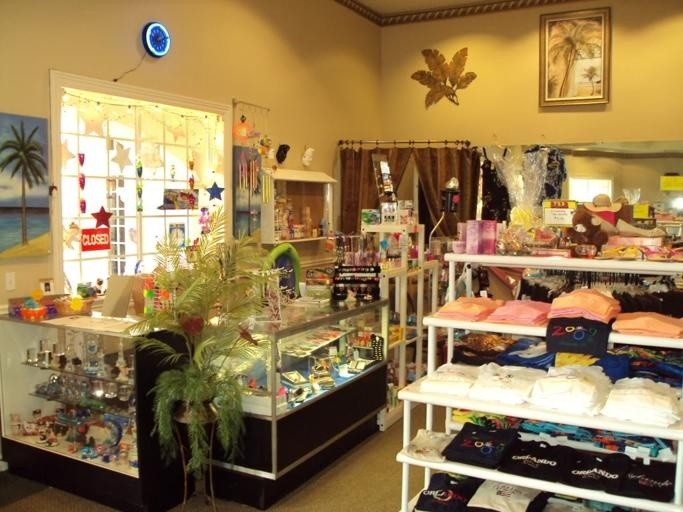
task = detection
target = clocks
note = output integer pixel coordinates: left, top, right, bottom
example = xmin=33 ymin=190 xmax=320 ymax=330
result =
xmin=142 ymin=18 xmax=172 ymax=60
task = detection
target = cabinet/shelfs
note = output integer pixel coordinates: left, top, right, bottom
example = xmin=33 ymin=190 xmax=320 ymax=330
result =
xmin=201 ymin=293 xmax=390 ymax=512
xmin=357 ymin=219 xmax=440 ymax=433
xmin=400 ymin=252 xmax=682 ymax=511
xmin=0 ymin=309 xmax=192 ymax=512
xmin=260 ymin=168 xmax=338 ymax=269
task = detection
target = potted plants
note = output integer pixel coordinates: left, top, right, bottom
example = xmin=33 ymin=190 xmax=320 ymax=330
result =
xmin=126 ymin=204 xmax=295 ymax=512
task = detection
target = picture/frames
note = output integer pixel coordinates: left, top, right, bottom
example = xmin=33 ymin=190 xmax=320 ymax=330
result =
xmin=535 ymin=6 xmax=611 ymax=111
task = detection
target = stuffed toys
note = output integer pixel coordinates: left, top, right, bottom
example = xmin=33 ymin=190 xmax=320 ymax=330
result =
xmin=571 ymin=211 xmax=608 ymax=258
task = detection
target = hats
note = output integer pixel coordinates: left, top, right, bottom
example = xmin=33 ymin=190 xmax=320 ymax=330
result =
xmin=584 ymin=194 xmax=622 ymax=213
